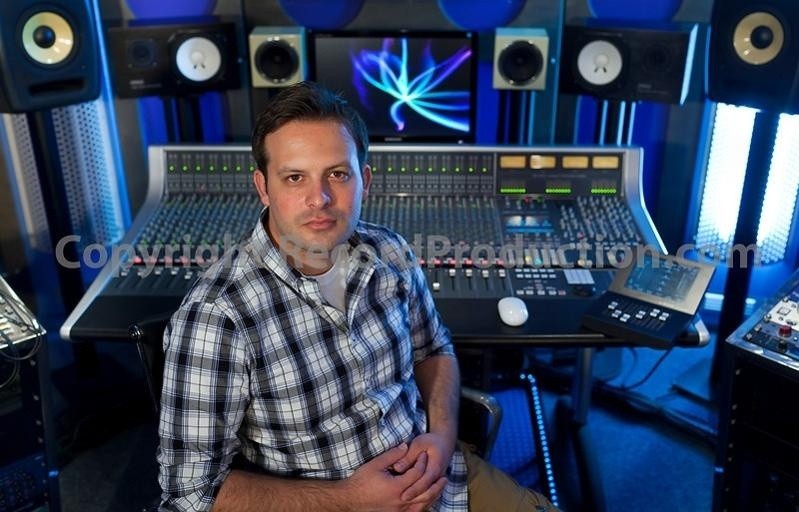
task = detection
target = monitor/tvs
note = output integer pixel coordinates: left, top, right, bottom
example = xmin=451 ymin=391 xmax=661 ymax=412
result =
xmin=306 ymin=29 xmax=479 ymax=143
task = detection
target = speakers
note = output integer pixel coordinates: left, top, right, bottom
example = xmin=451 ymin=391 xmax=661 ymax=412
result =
xmin=0 ymin=0 xmax=102 ymax=110
xmin=709 ymin=2 xmax=799 ymax=113
xmin=558 ymin=18 xmax=698 ymax=105
xmin=249 ymin=24 xmax=305 ymax=91
xmin=493 ymin=26 xmax=551 ymax=91
xmin=107 ymin=14 xmax=242 ymax=100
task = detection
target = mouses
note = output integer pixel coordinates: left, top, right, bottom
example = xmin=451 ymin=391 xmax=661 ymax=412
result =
xmin=497 ymin=297 xmax=529 ymax=327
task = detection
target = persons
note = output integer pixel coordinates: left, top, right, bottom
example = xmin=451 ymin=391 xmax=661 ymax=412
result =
xmin=152 ymin=79 xmax=559 ymax=512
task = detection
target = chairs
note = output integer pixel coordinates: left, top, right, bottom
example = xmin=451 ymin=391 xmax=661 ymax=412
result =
xmin=131 ymin=310 xmax=501 ymax=465
